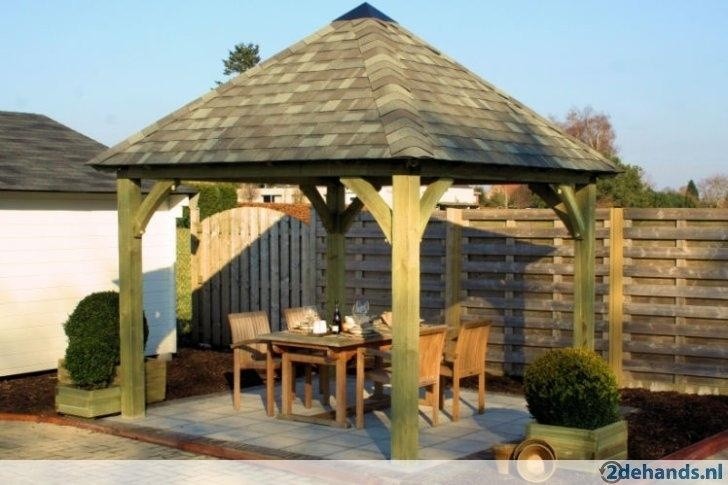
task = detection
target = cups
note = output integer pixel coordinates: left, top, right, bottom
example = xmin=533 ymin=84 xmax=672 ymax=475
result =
xmin=313 ymin=320 xmax=326 ymax=334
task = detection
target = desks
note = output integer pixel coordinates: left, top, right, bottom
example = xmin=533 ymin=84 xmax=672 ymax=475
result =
xmin=259 ymin=323 xmax=449 ymax=429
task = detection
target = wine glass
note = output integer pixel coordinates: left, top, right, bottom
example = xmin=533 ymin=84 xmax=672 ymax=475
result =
xmin=351 ymin=300 xmax=369 ymax=325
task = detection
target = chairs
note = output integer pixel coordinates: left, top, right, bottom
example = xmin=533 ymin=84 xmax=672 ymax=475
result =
xmin=228 ymin=310 xmax=312 ymax=417
xmin=284 ymin=305 xmax=330 ymax=394
xmin=439 ymin=319 xmax=492 ymax=421
xmin=355 ymin=326 xmax=447 ymax=429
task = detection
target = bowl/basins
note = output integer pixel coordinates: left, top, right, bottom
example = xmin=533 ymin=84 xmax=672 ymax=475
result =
xmin=517 ymin=444 xmax=555 ymax=483
xmin=491 ymin=444 xmax=516 ymax=473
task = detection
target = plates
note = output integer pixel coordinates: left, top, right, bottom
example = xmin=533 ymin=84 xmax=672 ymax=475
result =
xmin=298 ymin=326 xmax=329 ymax=331
xmin=346 ymin=328 xmax=372 ymax=334
xmin=513 ymin=439 xmax=556 ymax=462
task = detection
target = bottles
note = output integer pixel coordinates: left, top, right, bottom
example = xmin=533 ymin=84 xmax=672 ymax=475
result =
xmin=331 ymin=299 xmax=341 ymax=334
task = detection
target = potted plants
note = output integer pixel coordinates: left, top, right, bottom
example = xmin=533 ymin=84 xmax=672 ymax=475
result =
xmin=521 ymin=345 xmax=630 ymax=478
xmin=54 ymin=291 xmax=167 ymax=419
